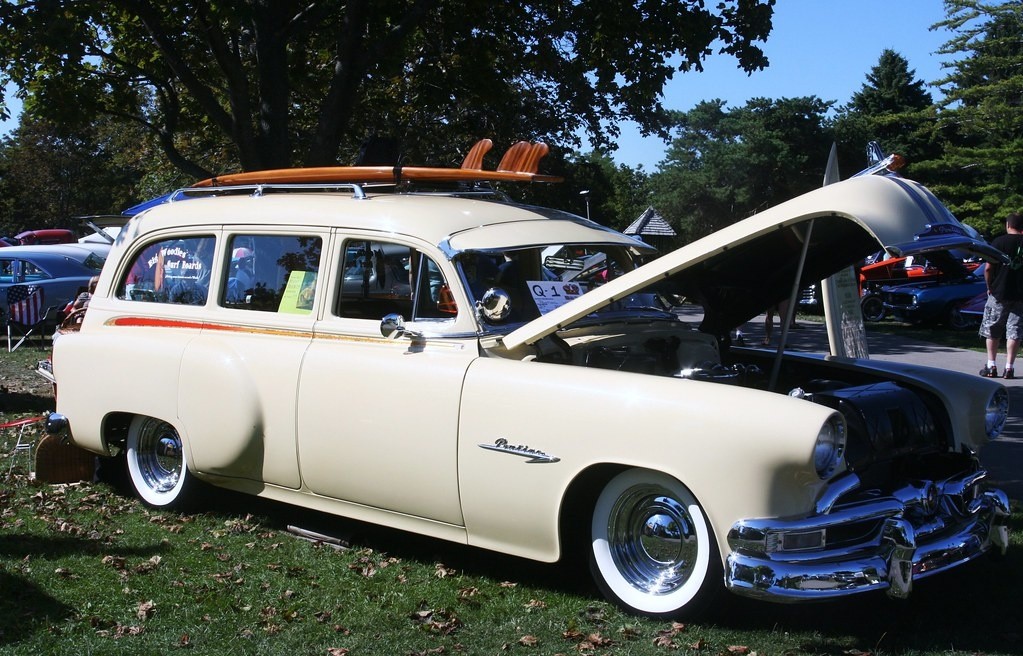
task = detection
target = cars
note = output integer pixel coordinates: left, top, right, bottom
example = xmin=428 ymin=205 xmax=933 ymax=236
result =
xmin=0 ymin=229 xmax=113 ymax=326
xmin=344 ymin=246 xmax=411 ymax=294
xmin=799 ymin=233 xmax=988 ymax=320
xmin=35 ymin=139 xmax=1010 ymax=615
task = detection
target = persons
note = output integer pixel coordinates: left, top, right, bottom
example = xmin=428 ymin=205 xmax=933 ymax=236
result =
xmin=230 ymin=259 xmax=253 ymax=287
xmin=71 ymin=275 xmax=100 ymax=315
xmin=125 ymin=240 xmax=208 ymax=302
xmin=978 ymin=212 xmax=1023 ymax=379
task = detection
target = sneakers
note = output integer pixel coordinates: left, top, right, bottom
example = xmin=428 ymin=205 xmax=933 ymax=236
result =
xmin=979 ymin=364 xmax=997 ymax=377
xmin=1003 ymin=368 xmax=1014 ymax=378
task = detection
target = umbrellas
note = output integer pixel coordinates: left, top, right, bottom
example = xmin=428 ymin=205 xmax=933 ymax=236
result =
xmin=232 ymin=247 xmax=253 ymax=259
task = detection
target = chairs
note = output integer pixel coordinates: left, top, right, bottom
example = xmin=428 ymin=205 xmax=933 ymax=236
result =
xmin=5 ymin=284 xmax=60 ymax=353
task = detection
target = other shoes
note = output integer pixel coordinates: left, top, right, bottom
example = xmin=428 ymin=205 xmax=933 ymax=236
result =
xmin=736 ymin=330 xmax=743 ymax=343
xmin=761 ymin=341 xmax=769 ymax=345
xmin=790 ymin=324 xmax=805 ymax=329
